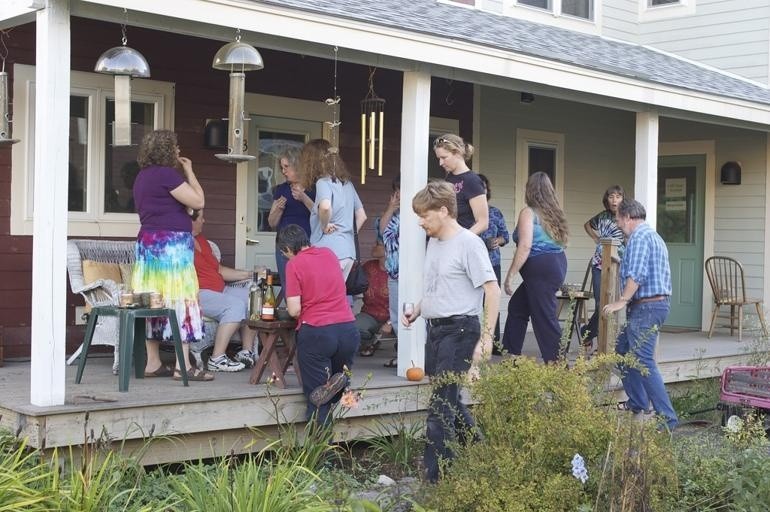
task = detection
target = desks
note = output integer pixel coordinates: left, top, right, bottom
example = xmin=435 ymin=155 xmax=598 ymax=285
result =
xmin=555 ymin=293 xmax=591 ymax=355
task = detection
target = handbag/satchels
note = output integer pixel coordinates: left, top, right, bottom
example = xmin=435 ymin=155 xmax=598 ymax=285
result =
xmin=345 ymin=208 xmax=370 ymax=296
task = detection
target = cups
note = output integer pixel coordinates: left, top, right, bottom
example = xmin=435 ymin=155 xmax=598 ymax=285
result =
xmin=575 ymin=291 xmax=584 ymax=296
xmin=119 ymin=292 xmax=165 ymax=309
xmin=487 ymin=237 xmax=495 ymax=249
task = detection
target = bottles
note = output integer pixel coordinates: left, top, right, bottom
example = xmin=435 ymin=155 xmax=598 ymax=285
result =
xmin=247 ymin=271 xmax=278 ymax=321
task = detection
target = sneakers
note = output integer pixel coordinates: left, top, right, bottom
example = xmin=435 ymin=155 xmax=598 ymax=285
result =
xmin=207 ymin=349 xmax=256 ymax=372
xmin=308 ymin=372 xmax=350 ymax=407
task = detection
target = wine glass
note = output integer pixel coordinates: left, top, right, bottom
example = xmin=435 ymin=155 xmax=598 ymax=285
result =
xmin=401 ymin=302 xmax=415 ymax=330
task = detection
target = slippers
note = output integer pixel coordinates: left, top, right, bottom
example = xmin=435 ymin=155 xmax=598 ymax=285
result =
xmin=384 ymin=358 xmax=397 ymax=368
xmin=359 ymin=339 xmax=381 ymax=357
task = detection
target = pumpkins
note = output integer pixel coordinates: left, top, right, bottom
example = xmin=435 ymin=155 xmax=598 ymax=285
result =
xmin=406 ymin=360 xmax=424 ymax=381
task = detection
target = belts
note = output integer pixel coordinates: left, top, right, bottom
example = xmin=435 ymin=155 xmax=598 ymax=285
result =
xmin=628 ymin=294 xmax=668 ymax=303
xmin=427 ymin=317 xmax=457 ymax=328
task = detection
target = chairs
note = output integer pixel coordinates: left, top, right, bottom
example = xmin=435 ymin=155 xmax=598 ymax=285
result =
xmin=704 ymin=255 xmax=770 ymax=343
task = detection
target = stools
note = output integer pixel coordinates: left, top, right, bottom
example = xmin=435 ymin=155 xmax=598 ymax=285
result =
xmin=75 ymin=304 xmax=190 ymax=394
xmin=241 ymin=313 xmax=304 ymax=390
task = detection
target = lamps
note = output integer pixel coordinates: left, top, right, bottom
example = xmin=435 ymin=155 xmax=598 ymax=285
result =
xmin=0 ymin=26 xmax=23 ymax=148
xmin=95 ymin=5 xmax=151 ymax=149
xmin=212 ymin=25 xmax=267 ymax=167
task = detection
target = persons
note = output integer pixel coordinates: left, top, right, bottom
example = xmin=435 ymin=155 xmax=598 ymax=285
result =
xmin=401 ymin=180 xmax=501 ymax=488
xmin=352 ymin=133 xmax=509 ymax=383
xmin=580 ymin=185 xmax=627 ymax=350
xmin=501 ymin=172 xmax=570 ymax=371
xmin=276 ymin=224 xmax=361 ymax=475
xmin=267 ymin=148 xmax=316 ymax=306
xmin=300 ymin=138 xmax=367 ymax=283
xmin=191 ymin=206 xmax=255 ymax=373
xmin=129 ymin=128 xmax=215 ymax=381
xmin=602 ymin=196 xmax=679 ymax=435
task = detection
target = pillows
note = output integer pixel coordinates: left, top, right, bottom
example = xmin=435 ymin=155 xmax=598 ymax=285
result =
xmin=80 ymin=259 xmax=124 ymax=301
xmin=118 ymin=262 xmax=131 ymax=279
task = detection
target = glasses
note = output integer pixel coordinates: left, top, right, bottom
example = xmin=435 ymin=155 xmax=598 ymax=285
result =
xmin=432 ymin=138 xmax=462 ymax=150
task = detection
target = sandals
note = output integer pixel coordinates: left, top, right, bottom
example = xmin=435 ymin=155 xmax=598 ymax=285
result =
xmin=616 ymin=402 xmax=651 ymax=415
xmin=144 ymin=361 xmax=216 ymax=381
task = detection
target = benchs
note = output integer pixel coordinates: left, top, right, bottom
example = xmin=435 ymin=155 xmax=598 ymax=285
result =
xmin=65 ymin=232 xmax=261 ymax=376
